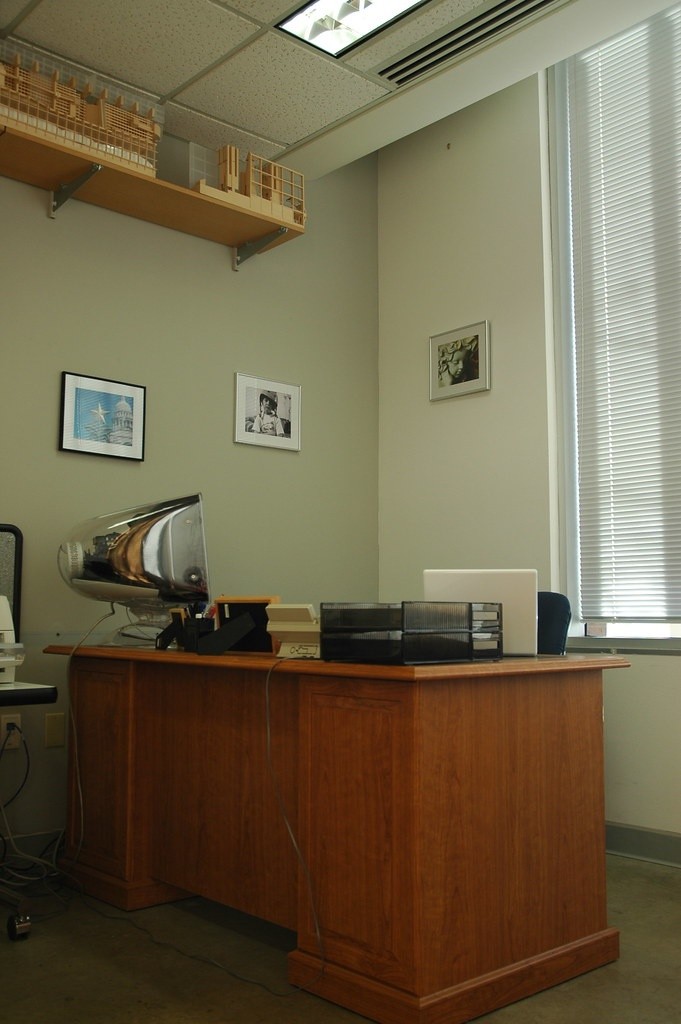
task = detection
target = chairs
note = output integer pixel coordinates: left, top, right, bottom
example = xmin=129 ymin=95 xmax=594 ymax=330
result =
xmin=538 ymin=592 xmax=574 ymax=656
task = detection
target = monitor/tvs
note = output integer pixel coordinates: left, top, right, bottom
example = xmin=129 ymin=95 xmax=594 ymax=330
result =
xmin=58 ymin=491 xmax=211 ymax=648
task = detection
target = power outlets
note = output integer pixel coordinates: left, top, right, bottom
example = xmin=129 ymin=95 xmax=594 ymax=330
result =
xmin=44 ymin=712 xmax=65 ymax=748
xmin=0 ymin=714 xmax=21 ymax=749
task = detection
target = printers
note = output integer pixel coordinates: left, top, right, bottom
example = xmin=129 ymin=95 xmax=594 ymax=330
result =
xmin=0 ymin=596 xmax=25 ymax=683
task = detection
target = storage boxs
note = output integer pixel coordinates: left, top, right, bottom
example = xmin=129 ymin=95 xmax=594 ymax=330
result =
xmin=320 ymin=601 xmax=505 ymax=667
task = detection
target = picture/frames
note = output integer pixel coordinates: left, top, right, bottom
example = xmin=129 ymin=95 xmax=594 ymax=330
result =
xmin=56 ymin=370 xmax=148 ymax=462
xmin=232 ymin=372 xmax=303 ymax=452
xmin=213 ymin=596 xmax=280 ymax=655
xmin=427 ymin=319 xmax=491 ymax=402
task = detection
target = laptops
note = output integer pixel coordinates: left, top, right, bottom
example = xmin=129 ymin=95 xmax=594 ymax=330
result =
xmin=422 ymin=568 xmax=539 ymax=657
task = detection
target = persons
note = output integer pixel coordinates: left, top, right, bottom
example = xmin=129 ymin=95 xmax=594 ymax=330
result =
xmin=252 ymin=390 xmax=284 ymax=437
xmin=438 ymin=348 xmax=470 ymax=387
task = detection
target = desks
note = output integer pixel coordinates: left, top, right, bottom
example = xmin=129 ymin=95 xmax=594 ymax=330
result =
xmin=42 ymin=644 xmax=633 ymax=1024
